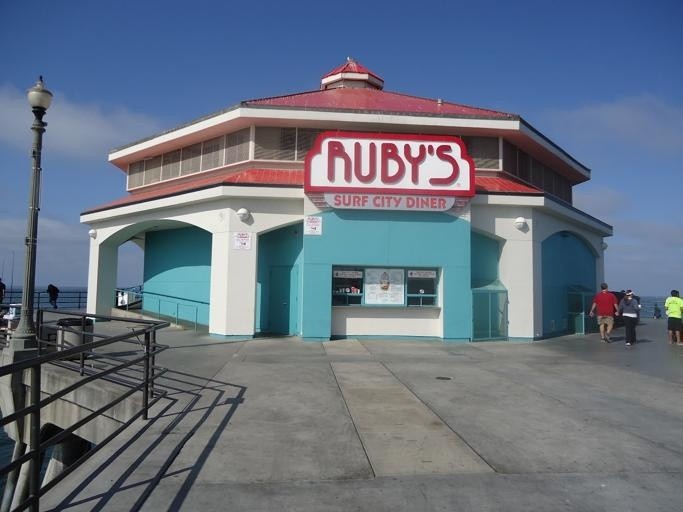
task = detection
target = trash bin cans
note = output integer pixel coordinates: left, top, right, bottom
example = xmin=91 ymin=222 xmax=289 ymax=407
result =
xmin=56 ymin=318 xmax=94 ymax=355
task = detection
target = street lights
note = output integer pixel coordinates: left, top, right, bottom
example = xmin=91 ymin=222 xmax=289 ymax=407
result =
xmin=15 ymin=75 xmax=53 ymax=334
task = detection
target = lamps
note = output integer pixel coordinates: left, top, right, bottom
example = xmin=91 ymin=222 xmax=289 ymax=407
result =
xmin=514 ymin=216 xmax=529 ymax=231
xmin=236 ymin=207 xmax=251 ymax=223
xmin=87 ymin=229 xmax=97 ymax=239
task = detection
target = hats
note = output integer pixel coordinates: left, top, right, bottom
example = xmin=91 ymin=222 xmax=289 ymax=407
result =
xmin=626 ymin=290 xmax=635 ymax=295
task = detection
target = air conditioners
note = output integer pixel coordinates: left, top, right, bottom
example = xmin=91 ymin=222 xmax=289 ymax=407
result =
xmin=116 ymin=286 xmax=141 ymax=307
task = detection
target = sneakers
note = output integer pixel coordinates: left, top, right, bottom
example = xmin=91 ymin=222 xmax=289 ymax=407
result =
xmin=668 ymin=340 xmax=683 ymax=345
xmin=601 ymin=333 xmax=611 ymax=343
xmin=625 ymin=342 xmax=631 ymax=346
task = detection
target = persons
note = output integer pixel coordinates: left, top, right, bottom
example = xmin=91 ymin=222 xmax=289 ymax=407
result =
xmin=589 ymin=283 xmax=619 ymax=343
xmin=664 ymin=290 xmax=683 ymax=345
xmin=652 ymin=304 xmax=661 ymax=319
xmin=617 ymin=289 xmax=641 ymax=346
xmin=0 ymin=277 xmax=6 ymax=304
xmin=46 ymin=283 xmax=59 ymax=309
